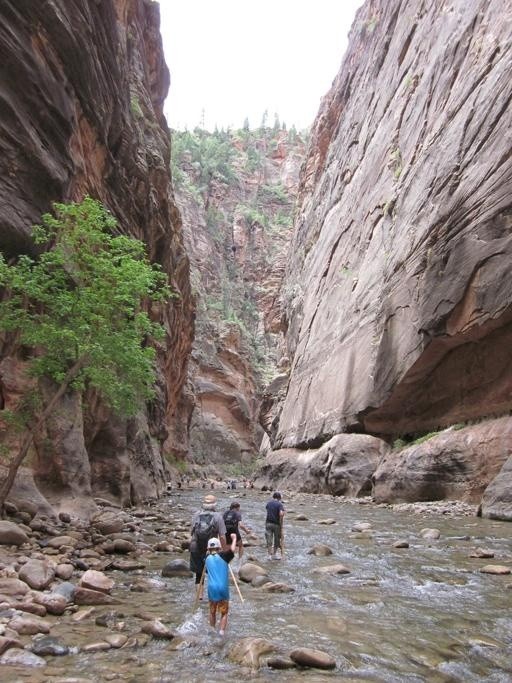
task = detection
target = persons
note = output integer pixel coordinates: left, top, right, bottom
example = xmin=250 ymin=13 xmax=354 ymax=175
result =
xmin=176 ymin=473 xmax=255 ymax=491
xmin=190 ymin=495 xmax=227 ymax=601
xmin=263 ymin=491 xmax=285 ymax=562
xmin=221 ymin=501 xmax=251 ymax=561
xmin=205 ymin=532 xmax=237 ymax=636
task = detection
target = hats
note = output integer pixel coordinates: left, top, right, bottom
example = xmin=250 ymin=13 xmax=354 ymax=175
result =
xmin=204 ymin=495 xmax=217 ymax=504
xmin=206 ymin=537 xmax=222 ymax=550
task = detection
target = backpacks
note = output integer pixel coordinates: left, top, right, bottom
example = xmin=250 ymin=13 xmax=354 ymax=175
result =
xmin=192 ymin=511 xmax=220 ymax=548
xmin=225 ymin=511 xmax=238 ymax=533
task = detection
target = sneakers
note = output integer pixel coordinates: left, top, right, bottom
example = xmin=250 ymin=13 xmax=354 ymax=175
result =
xmin=266 ymin=554 xmax=282 ymax=561
xmin=219 ymin=630 xmax=225 ymax=636
